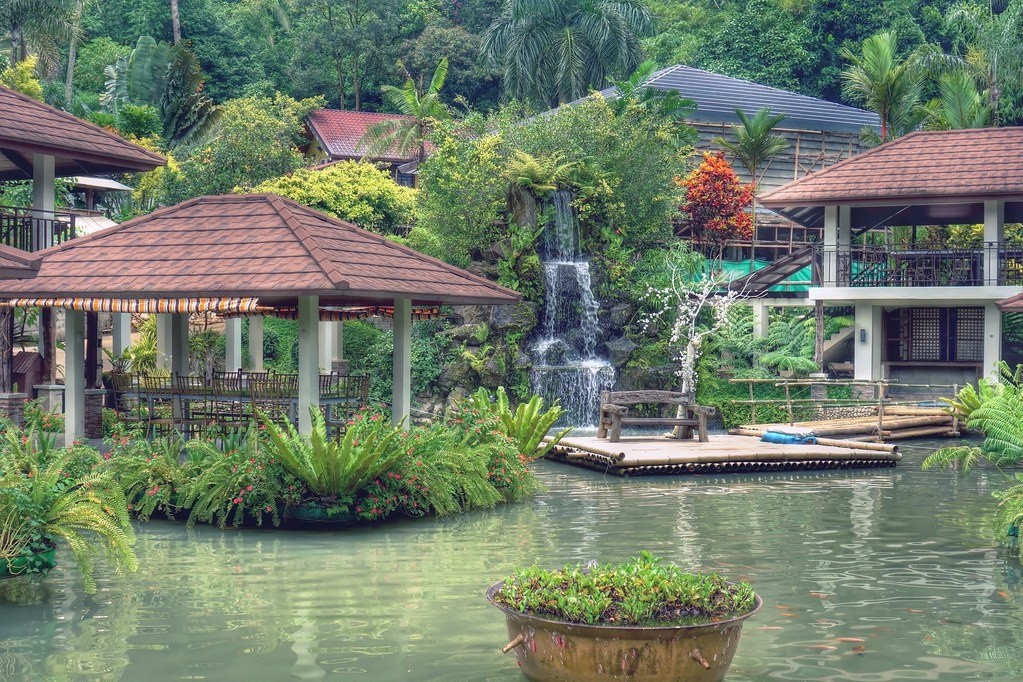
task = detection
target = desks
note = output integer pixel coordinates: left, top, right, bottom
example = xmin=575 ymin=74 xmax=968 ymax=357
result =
xmin=123 ymin=389 xmax=358 ymax=449
xmin=887 ymin=249 xmax=1023 ymax=285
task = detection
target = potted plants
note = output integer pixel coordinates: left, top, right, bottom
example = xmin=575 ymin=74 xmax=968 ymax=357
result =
xmin=0 ymin=386 xmax=763 ymax=682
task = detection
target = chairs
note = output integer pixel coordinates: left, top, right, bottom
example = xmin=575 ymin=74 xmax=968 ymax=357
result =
xmin=111 ymin=368 xmax=370 ymax=454
xmin=860 ymin=240 xmax=1023 ymax=285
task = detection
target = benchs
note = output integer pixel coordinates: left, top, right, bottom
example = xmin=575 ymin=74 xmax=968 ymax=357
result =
xmin=597 ymin=390 xmax=716 ymax=442
